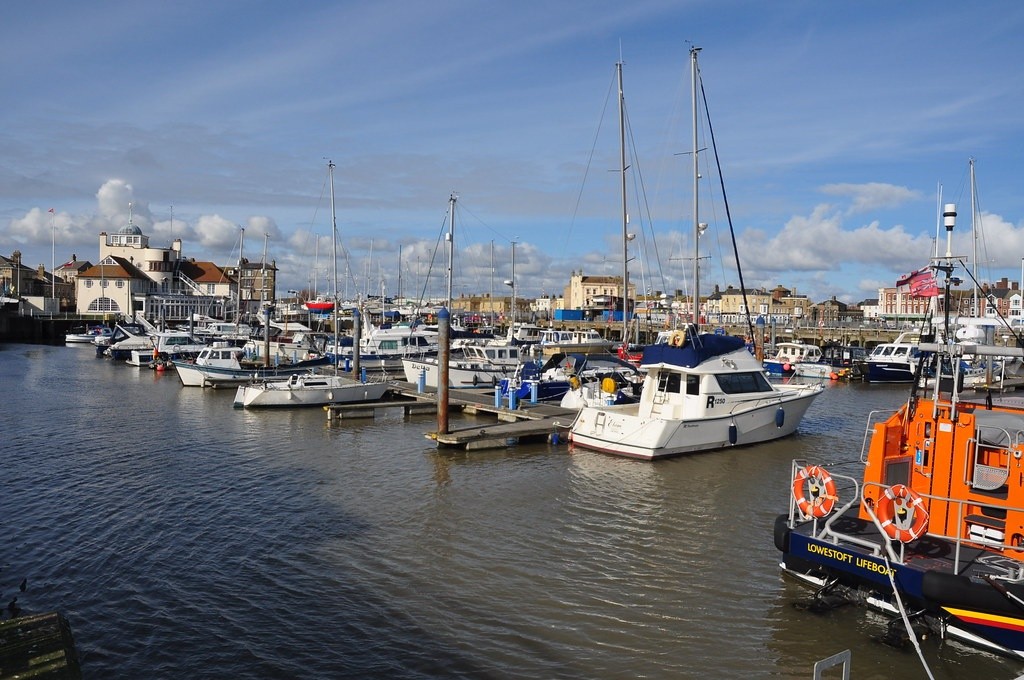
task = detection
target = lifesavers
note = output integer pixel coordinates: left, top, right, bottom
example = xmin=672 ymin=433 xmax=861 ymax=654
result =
xmin=667 ymin=331 xmax=686 ymax=348
xmin=792 ymin=466 xmax=839 ymax=517
xmin=876 ymin=484 xmax=932 ymax=543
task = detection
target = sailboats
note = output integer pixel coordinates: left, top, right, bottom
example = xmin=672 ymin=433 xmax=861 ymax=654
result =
xmin=64 ymin=26 xmax=1024 ymax=463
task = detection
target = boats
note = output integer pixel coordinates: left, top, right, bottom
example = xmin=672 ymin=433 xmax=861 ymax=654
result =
xmin=773 ymin=181 xmax=1024 ymax=662
